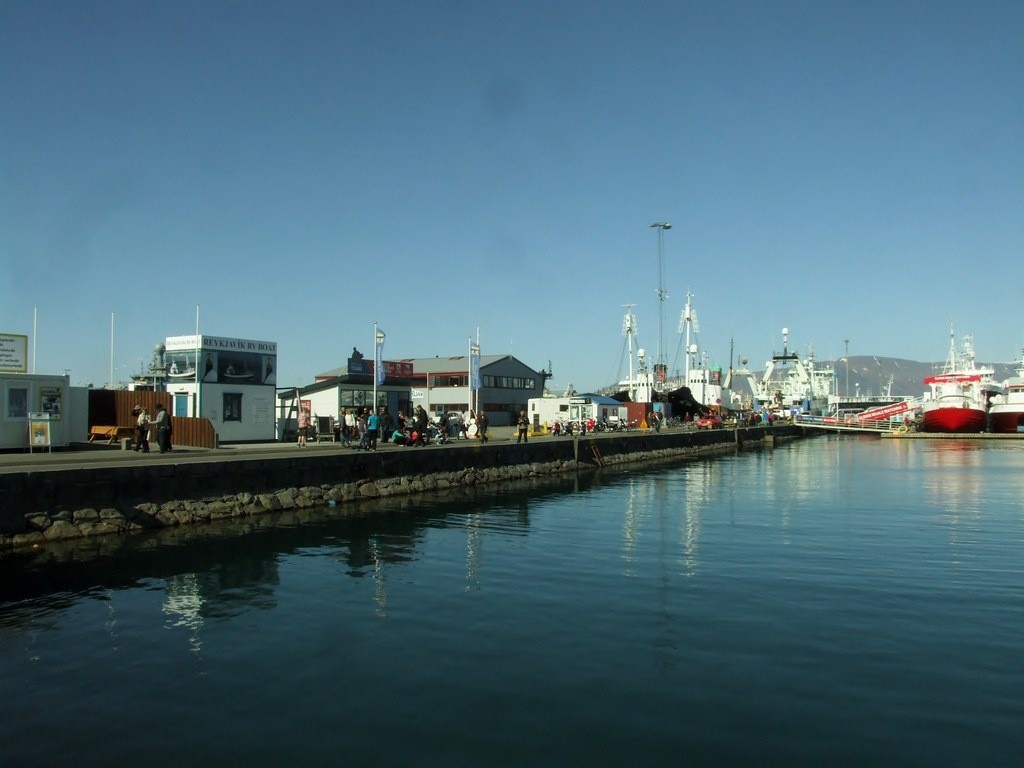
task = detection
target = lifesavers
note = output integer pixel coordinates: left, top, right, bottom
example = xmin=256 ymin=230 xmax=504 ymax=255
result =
xmin=845 ymin=417 xmax=854 ymax=424
xmin=898 ymin=425 xmax=908 ymax=434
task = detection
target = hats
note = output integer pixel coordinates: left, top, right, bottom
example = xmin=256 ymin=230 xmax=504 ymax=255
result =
xmin=155 ymin=403 xmax=162 ymax=409
xmin=135 ymin=405 xmax=141 ymax=410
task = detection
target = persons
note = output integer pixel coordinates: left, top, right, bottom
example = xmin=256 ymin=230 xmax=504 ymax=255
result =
xmin=338 ymin=405 xmax=469 ymax=451
xmin=647 ymin=409 xmax=663 ymax=434
xmin=479 ymin=411 xmax=488 ymax=444
xmin=131 ymin=406 xmax=151 ymax=453
xmin=684 ymin=409 xmax=774 ymax=431
xmin=904 ymin=415 xmax=909 ymax=426
xmin=148 ymin=404 xmax=173 ymax=454
xmin=517 ymin=411 xmax=529 ymax=443
xmin=297 ymin=407 xmax=309 ymax=448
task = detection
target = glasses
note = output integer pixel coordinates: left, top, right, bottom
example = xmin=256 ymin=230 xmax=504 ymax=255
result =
xmin=364 ymin=410 xmax=368 ymax=411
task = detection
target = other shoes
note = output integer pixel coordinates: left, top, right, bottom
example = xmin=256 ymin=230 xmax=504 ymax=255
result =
xmin=133 ymin=447 xmax=139 ymax=451
xmin=142 ymin=449 xmax=149 ymax=453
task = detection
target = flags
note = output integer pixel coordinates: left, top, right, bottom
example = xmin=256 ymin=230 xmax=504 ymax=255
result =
xmin=470 ymin=341 xmax=481 ymax=391
xmin=376 ymin=329 xmax=385 ymax=385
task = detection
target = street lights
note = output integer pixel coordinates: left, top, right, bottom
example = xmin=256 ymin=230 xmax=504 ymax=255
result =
xmin=650 ymin=222 xmax=670 ymax=403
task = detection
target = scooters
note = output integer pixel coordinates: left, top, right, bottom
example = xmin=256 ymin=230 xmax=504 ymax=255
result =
xmin=550 ymin=417 xmax=641 ymax=436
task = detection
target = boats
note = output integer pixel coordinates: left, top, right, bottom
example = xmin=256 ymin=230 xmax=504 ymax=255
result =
xmin=921 ymin=319 xmax=988 ymax=432
xmin=746 ymin=327 xmax=839 ymax=416
xmin=987 ymin=349 xmax=1024 ymax=433
xmin=671 ymin=286 xmax=744 ymax=411
xmin=608 ymin=302 xmax=664 ymax=401
